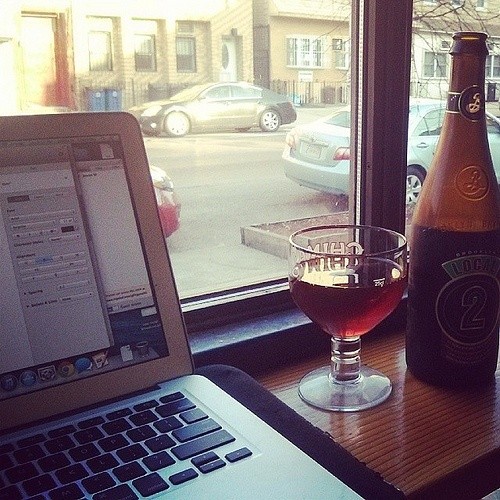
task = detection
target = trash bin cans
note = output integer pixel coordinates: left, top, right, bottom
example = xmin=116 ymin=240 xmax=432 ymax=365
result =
xmin=85 ymin=86 xmax=105 ymax=112
xmin=105 ymin=87 xmax=122 ymax=111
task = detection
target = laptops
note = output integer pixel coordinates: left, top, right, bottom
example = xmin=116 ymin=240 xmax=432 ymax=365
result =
xmin=0 ymin=112 xmax=409 ymax=500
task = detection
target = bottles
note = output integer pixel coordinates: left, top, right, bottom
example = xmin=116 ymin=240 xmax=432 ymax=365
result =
xmin=407 ymin=32 xmax=500 ymax=383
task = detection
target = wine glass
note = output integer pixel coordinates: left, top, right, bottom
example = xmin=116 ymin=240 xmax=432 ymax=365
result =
xmin=289 ymin=225 xmax=407 ymax=411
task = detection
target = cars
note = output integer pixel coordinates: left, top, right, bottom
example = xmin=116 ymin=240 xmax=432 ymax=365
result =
xmin=282 ymin=97 xmax=500 ymax=225
xmin=149 ymin=165 xmax=182 ymax=238
xmin=127 ymin=81 xmax=297 ymax=138
